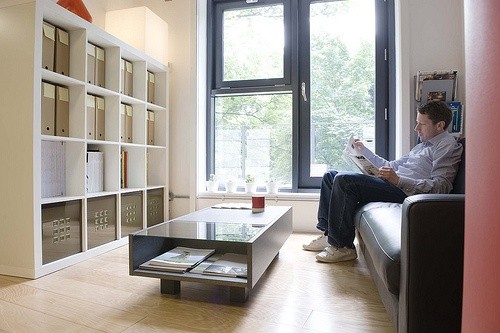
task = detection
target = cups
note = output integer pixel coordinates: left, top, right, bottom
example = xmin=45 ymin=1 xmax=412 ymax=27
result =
xmin=251 ymin=195 xmax=265 ymax=212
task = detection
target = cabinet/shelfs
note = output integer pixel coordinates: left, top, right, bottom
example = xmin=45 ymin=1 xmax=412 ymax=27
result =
xmin=1 ymin=0 xmax=171 ymax=279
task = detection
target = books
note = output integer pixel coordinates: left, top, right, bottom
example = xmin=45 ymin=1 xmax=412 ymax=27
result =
xmin=40 ymin=141 xmax=128 ymax=199
xmin=138 ymin=246 xmax=248 ymax=278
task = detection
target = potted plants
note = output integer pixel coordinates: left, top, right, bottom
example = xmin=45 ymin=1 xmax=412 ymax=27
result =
xmin=244 ymin=174 xmax=256 ymax=193
xmin=206 ymin=174 xmax=219 ymax=192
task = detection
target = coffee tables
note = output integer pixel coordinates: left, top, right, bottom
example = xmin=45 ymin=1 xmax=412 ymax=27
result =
xmin=128 ymin=203 xmax=293 ymax=306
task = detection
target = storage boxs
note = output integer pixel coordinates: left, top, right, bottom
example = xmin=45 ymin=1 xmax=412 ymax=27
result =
xmin=121 ymin=191 xmax=143 ymax=237
xmin=87 ymin=195 xmax=117 ymax=249
xmin=147 ymin=188 xmax=164 ymax=228
xmin=42 ymin=200 xmax=81 ymax=265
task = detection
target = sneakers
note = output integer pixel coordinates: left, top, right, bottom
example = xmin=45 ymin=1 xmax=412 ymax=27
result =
xmin=303 ymin=233 xmax=328 ymax=251
xmin=315 ymin=244 xmax=357 ymax=263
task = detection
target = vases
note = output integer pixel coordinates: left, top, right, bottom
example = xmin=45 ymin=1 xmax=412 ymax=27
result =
xmin=226 ymin=182 xmax=237 ymax=192
xmin=266 ymin=183 xmax=278 ymax=194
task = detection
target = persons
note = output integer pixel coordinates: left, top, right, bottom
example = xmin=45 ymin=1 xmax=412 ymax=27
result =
xmin=303 ymin=100 xmax=463 ymax=264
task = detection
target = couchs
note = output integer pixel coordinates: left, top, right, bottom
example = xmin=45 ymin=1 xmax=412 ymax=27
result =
xmin=355 ymin=138 xmax=465 ymax=333
